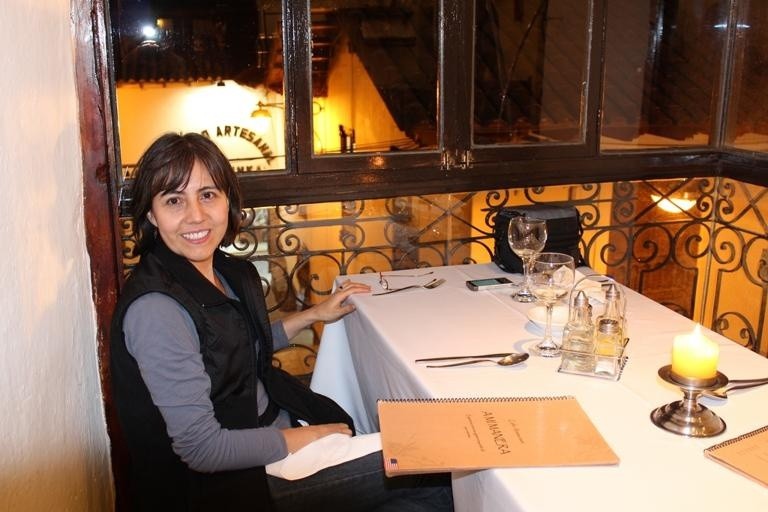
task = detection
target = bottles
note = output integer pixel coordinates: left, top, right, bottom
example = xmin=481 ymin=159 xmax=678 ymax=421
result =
xmin=564 ymin=283 xmax=623 ymax=372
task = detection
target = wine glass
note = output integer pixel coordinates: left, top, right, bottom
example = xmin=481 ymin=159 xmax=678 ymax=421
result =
xmin=506 ymin=217 xmax=576 ymax=358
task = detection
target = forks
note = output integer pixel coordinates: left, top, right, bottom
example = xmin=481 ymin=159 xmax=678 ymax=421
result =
xmin=370 ymin=277 xmax=446 ymax=297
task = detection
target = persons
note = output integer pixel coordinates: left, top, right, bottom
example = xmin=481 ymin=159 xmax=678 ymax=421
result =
xmin=106 ymin=128 xmax=454 ymax=512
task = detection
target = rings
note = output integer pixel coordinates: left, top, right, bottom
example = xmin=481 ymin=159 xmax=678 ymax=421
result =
xmin=339 ymin=286 xmax=342 ymax=289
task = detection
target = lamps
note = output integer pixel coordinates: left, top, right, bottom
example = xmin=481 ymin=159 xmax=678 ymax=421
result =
xmin=649 ymin=180 xmax=702 ymax=213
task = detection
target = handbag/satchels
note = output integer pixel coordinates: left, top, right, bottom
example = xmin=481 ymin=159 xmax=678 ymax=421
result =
xmin=486 ymin=200 xmax=584 ymax=274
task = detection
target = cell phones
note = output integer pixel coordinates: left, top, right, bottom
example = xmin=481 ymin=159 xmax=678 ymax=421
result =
xmin=466 ymin=275 xmax=512 ymax=291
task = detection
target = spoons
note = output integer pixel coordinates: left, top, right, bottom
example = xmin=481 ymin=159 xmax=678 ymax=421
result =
xmin=427 ymin=354 xmax=530 ymax=367
xmin=697 ymin=378 xmax=768 ymax=399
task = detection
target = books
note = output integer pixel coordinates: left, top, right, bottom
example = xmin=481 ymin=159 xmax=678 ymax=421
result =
xmin=374 ymin=395 xmax=620 ymax=481
xmin=701 ymin=424 xmax=768 ymax=490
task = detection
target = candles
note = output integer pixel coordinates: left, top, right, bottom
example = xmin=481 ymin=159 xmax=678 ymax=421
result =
xmin=672 ymin=324 xmax=720 ymax=386
